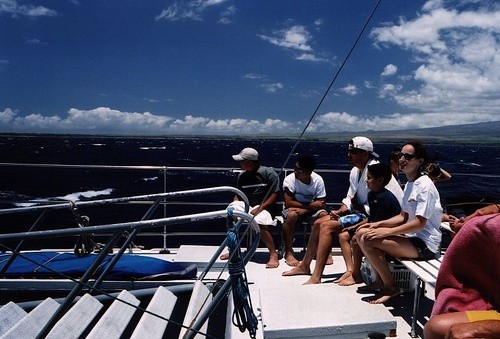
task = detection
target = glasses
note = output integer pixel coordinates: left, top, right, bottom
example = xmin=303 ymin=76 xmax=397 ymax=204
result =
xmin=398 ymin=153 xmax=417 ymax=161
xmin=293 ymin=164 xmax=305 ymax=174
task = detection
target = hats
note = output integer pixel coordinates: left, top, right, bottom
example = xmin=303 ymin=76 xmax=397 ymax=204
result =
xmin=232 ymin=148 xmax=259 ymax=161
xmin=349 ymin=136 xmax=379 ymax=158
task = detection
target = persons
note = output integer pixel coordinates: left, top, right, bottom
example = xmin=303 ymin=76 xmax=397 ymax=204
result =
xmin=334 ymin=163 xmax=401 ymax=286
xmin=282 ymin=136 xmax=404 ymax=285
xmin=220 ymin=147 xmax=280 ymax=268
xmin=387 ymin=145 xmax=459 ymax=222
xmin=283 ymin=152 xmax=333 ymax=265
xmin=355 ymin=142 xmax=443 ymax=304
